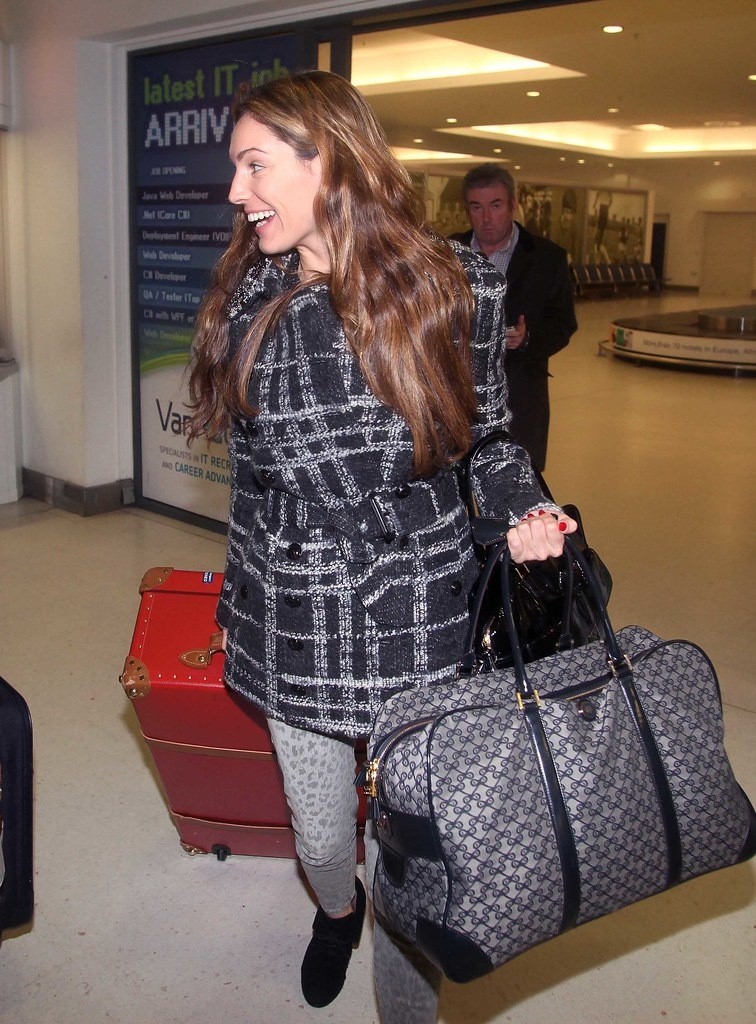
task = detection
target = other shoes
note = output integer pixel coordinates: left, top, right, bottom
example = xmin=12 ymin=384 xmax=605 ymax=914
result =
xmin=301 ymin=875 xmax=366 ymax=1008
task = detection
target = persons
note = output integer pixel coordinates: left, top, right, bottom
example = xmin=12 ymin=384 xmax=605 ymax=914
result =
xmin=447 ymin=162 xmax=578 ymax=471
xmin=206 ymin=72 xmax=580 ymax=1024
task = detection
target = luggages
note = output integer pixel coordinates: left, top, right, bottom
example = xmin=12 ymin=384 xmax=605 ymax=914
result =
xmin=0 ymin=676 xmax=34 ymax=928
xmin=120 ymin=565 xmax=369 ymax=867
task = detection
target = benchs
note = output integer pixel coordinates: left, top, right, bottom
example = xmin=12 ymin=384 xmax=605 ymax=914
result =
xmin=571 ymin=263 xmax=672 ymax=297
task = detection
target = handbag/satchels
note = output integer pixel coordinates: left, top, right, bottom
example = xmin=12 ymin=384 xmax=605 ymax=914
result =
xmin=458 ymin=430 xmax=614 ymax=676
xmin=353 ymin=534 xmax=756 ymax=984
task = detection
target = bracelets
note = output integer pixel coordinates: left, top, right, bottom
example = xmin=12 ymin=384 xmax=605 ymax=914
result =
xmin=509 ymin=502 xmax=564 ymax=524
